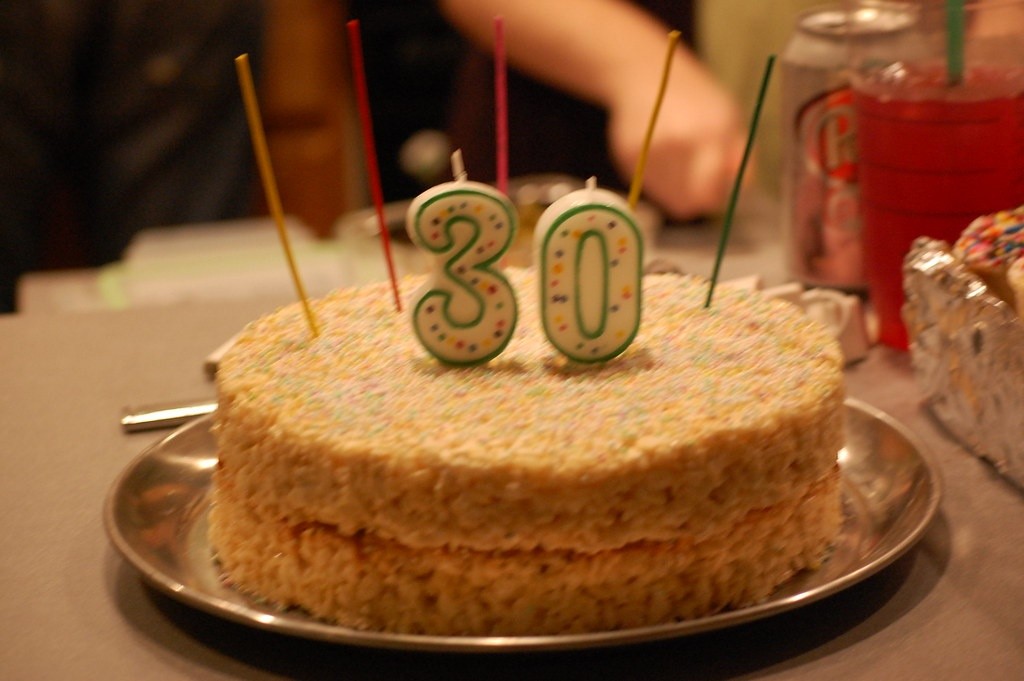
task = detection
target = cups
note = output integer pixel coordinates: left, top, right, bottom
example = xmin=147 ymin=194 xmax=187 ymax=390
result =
xmin=846 ymin=0 xmax=1024 ymax=352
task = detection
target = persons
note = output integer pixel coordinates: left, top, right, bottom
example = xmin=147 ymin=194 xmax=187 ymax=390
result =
xmin=424 ymin=1 xmax=758 ymax=225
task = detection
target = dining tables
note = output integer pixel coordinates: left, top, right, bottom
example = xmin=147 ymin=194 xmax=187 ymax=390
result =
xmin=0 ymin=197 xmax=1024 ymax=681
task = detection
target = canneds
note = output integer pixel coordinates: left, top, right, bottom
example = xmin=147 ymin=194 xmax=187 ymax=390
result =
xmin=780 ymin=4 xmax=924 ymax=298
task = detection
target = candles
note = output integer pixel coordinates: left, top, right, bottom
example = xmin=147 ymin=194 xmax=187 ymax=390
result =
xmin=404 ymin=147 xmax=518 ymax=368
xmin=533 ymin=175 xmax=648 ymax=365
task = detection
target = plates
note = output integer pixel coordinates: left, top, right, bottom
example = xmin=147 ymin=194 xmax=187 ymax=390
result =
xmin=99 ymin=393 xmax=942 ymax=650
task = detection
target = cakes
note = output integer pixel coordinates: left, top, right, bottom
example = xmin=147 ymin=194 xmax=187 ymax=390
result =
xmin=208 ymin=154 xmax=845 ymax=638
xmin=949 ymin=198 xmax=1024 ymax=323
